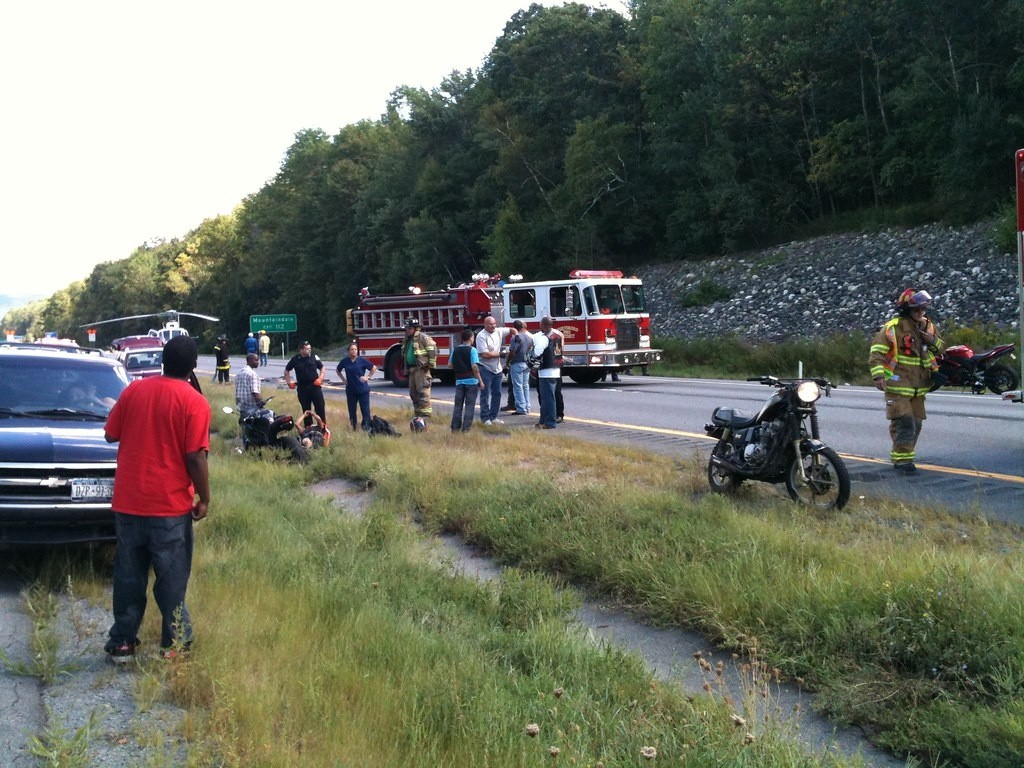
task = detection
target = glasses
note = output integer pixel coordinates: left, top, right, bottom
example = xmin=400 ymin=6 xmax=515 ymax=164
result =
xmin=913 ymin=306 xmax=926 ymax=312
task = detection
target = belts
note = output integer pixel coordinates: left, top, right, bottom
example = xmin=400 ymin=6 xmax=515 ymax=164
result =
xmin=408 ymin=364 xmax=416 ymax=367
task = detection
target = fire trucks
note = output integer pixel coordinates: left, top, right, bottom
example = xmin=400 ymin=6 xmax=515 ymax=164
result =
xmin=347 ymin=270 xmax=664 ymax=388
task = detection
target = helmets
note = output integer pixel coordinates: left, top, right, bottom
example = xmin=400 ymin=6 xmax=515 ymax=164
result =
xmin=402 ymin=318 xmax=418 ymax=328
xmin=410 ymin=417 xmax=427 ymax=434
xmin=898 ymin=288 xmax=927 ymax=307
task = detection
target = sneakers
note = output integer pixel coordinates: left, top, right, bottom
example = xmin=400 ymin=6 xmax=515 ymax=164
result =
xmin=109 ymin=645 xmax=133 ymax=663
xmin=161 ymin=644 xmax=188 ymax=659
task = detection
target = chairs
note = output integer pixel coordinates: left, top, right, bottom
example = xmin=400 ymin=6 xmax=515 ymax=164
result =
xmin=128 ymin=357 xmax=141 ymax=368
xmin=574 ymin=297 xmax=594 ymax=316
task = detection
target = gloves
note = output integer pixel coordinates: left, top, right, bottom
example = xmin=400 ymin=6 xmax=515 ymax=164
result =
xmin=313 ymin=379 xmax=322 ymax=386
xmin=289 ymin=383 xmax=297 ymax=389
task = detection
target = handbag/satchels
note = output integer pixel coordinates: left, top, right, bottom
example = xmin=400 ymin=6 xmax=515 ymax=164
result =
xmin=369 ymin=416 xmax=397 ymax=436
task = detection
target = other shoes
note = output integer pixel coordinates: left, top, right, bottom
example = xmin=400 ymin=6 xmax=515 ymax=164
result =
xmin=642 ymin=373 xmax=650 ymax=376
xmin=501 ymin=404 xmax=516 ymax=411
xmin=602 ymin=379 xmax=606 ymax=381
xmin=625 ymin=371 xmax=633 ymax=375
xmin=492 ymin=419 xmax=504 ymax=425
xmin=557 ymin=416 xmax=563 ymax=422
xmin=483 ymin=419 xmax=493 ymax=426
xmin=901 ymin=463 xmax=919 ymax=476
xmin=893 ymin=464 xmax=900 ymax=469
xmin=612 ymin=379 xmax=621 ymax=381
xmin=511 ymin=412 xmax=526 ymax=415
xmin=535 ymin=423 xmax=554 ymax=429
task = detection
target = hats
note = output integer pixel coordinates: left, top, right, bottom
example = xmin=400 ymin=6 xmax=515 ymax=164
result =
xmin=218 ymin=334 xmax=229 ymax=342
xmin=261 ymin=330 xmax=266 ymax=335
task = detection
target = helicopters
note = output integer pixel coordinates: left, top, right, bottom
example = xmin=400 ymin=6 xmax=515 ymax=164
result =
xmin=79 ymin=310 xmax=220 ymax=344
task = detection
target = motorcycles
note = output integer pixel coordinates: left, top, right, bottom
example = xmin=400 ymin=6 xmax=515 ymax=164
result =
xmin=703 ymin=375 xmax=850 ymax=511
xmin=927 ymin=343 xmax=1018 ymax=395
xmin=222 ymin=396 xmax=308 ymax=464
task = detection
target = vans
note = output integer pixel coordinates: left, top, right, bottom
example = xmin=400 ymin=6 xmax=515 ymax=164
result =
xmin=111 ymin=336 xmax=166 ymax=380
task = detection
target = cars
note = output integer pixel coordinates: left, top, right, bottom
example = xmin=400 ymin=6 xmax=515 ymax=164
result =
xmin=0 ymin=341 xmax=133 ymax=541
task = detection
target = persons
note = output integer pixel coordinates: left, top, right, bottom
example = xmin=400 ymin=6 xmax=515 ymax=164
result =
xmin=869 ymin=286 xmax=946 ymax=471
xmin=214 ymin=317 xmax=566 ymax=450
xmin=603 ymin=363 xmax=651 ymax=384
xmin=103 ymin=336 xmax=211 ymax=667
